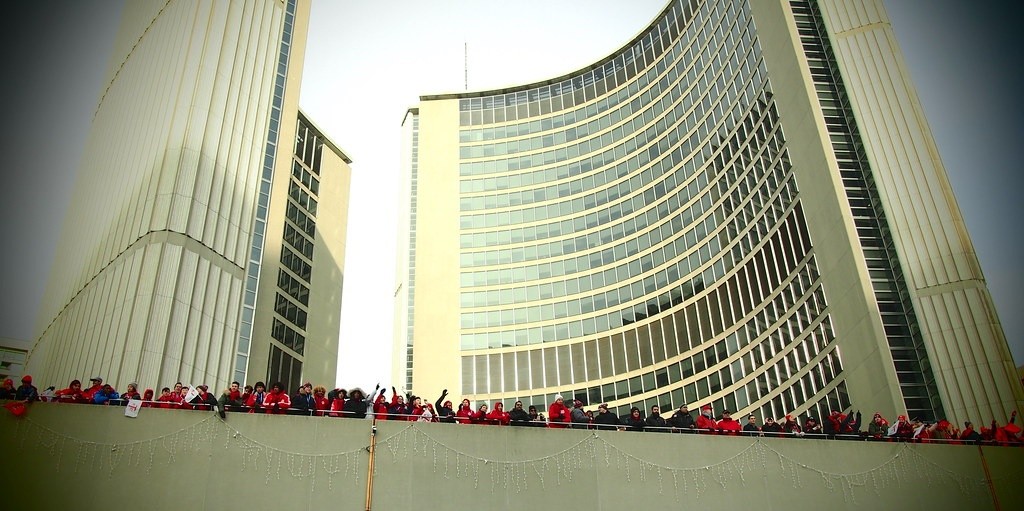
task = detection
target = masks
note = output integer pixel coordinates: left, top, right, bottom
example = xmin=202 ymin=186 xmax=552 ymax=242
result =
xmin=316 ymin=392 xmax=323 ymax=396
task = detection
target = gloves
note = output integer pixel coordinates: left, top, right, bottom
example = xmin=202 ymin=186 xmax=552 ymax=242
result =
xmin=273 ymin=404 xmax=279 ymax=411
xmin=1012 ymin=411 xmax=1016 ymax=416
xmin=375 ymin=383 xmax=451 ymax=409
xmin=226 ymin=400 xmax=235 ymax=405
xmin=220 ymin=411 xmax=225 ymax=418
xmin=133 ymin=395 xmax=141 ymax=399
xmin=459 ymin=404 xmax=463 ymax=410
xmin=334 ymin=388 xmax=339 ymax=392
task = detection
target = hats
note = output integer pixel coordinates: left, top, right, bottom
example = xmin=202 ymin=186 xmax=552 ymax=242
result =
xmin=598 ymin=403 xmax=608 ymax=409
xmin=197 ymin=385 xmax=208 ymax=392
xmin=302 ymin=381 xmax=312 ymax=390
xmin=21 ymin=375 xmax=32 ymax=383
xmin=89 ymin=377 xmax=102 ymax=382
xmin=679 ymin=403 xmax=732 ymax=414
xmin=128 ymin=383 xmax=138 ymax=390
xmin=555 ymin=394 xmax=563 ymax=402
xmin=573 ymin=399 xmax=583 ymax=408
xmin=3 ymin=379 xmax=14 ymax=385
xmin=529 ymin=406 xmax=535 ymax=411
xmin=630 ymin=407 xmax=640 ymax=414
xmin=765 ymin=409 xmax=990 ymax=433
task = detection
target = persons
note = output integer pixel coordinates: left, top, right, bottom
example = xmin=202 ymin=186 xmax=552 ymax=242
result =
xmin=1 ymin=372 xmax=1023 ymax=448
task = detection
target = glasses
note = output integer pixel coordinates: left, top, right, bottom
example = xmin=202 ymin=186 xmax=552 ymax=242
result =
xmin=531 ymin=410 xmax=536 ymax=412
